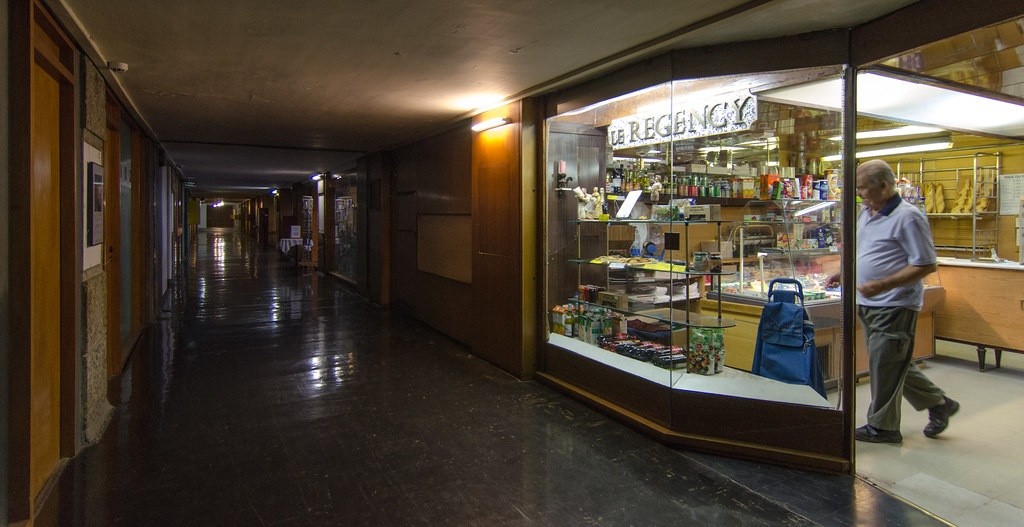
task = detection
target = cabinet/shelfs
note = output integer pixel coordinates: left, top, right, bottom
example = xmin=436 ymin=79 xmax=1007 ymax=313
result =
xmin=555 ymin=215 xmax=736 ymax=375
xmin=894 ymin=150 xmax=1002 ymax=262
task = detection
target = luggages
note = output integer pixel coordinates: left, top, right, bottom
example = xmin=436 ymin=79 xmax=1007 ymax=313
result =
xmin=752 ymin=278 xmax=827 ymax=401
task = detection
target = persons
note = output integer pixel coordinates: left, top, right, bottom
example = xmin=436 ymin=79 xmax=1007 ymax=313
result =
xmin=824 ymin=158 xmax=960 ymax=444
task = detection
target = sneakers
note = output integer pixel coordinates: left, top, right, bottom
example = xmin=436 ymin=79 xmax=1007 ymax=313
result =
xmin=855 ymin=424 xmax=903 ymax=443
xmin=923 ymin=395 xmax=960 ymax=436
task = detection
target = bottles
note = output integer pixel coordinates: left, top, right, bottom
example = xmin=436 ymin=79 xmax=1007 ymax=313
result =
xmin=607 ymin=162 xmax=651 ymax=194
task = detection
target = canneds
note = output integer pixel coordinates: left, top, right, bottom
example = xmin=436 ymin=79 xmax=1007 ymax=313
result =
xmin=665 ymin=173 xmax=733 ymax=198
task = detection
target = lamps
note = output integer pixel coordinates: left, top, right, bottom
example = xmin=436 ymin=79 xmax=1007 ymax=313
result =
xmin=467 ymin=116 xmax=513 ymax=135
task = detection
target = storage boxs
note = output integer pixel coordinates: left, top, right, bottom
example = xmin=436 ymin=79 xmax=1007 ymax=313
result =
xmin=707 ymin=197 xmax=948 ymax=391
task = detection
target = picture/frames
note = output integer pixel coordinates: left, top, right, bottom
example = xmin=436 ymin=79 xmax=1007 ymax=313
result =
xmin=86 ymin=159 xmax=106 ymax=248
xmin=290 ymin=224 xmax=302 ymax=240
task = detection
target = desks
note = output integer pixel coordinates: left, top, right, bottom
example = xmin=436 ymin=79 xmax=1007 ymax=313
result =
xmin=279 ymin=237 xmax=305 ymax=266
